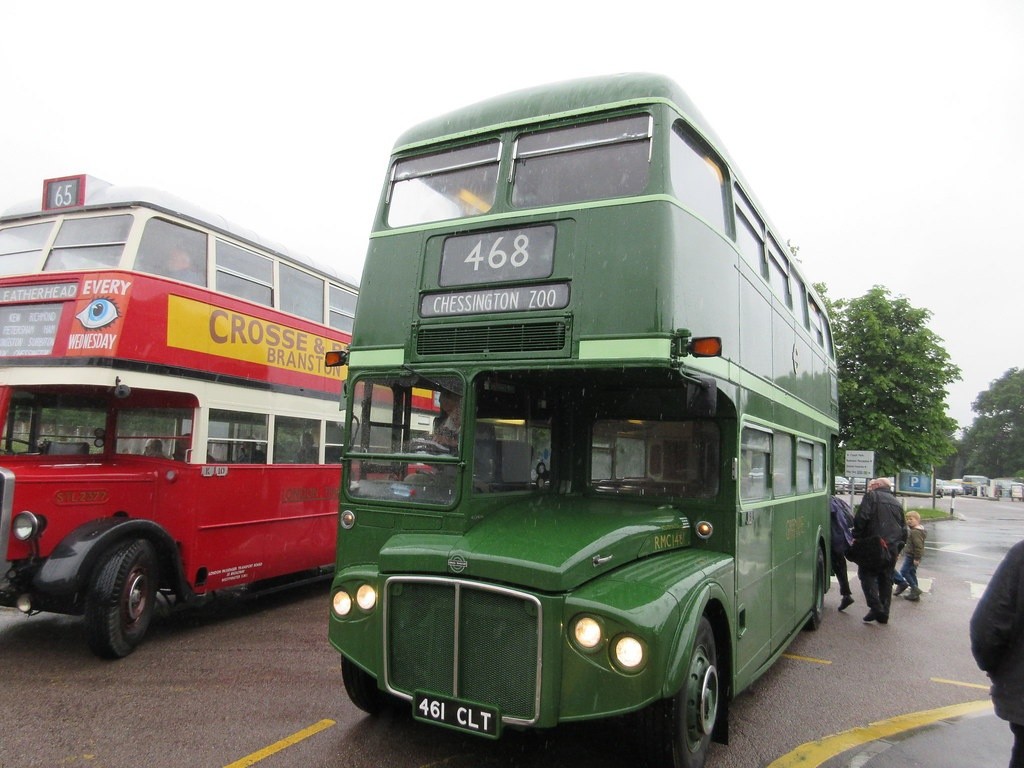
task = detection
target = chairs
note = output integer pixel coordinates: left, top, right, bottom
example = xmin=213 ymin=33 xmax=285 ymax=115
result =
xmin=592 ymin=478 xmax=685 ymax=498
xmin=44 ymin=440 xmax=89 ymax=455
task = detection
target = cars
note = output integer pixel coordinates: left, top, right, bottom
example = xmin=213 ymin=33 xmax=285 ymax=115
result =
xmin=835 ymin=474 xmax=1024 ymax=503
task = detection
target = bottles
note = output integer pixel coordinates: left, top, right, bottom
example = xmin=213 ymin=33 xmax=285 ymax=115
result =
xmin=391 ymin=483 xmax=415 ymax=497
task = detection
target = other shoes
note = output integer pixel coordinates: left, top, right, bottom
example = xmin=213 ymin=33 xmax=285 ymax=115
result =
xmin=894 ymin=581 xmax=908 ymax=596
xmin=864 ymin=606 xmax=884 ymax=621
xmin=838 ymin=597 xmax=854 ymax=611
xmin=876 ymin=613 xmax=889 ymax=623
xmin=905 ymin=588 xmax=923 ymax=601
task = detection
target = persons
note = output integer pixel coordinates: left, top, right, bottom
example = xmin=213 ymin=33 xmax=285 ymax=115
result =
xmin=237 ymin=437 xmax=265 ymax=463
xmin=970 ymin=539 xmax=1024 ymax=768
xmin=851 ymin=478 xmax=908 ymax=624
xmin=900 ymin=511 xmax=926 ymax=601
xmin=293 ymin=432 xmax=318 ymax=464
xmin=432 ymin=391 xmax=461 ymax=451
xmin=937 ymin=488 xmax=944 ymax=497
xmin=830 ymin=494 xmax=854 ymax=610
xmin=142 ymin=433 xmax=216 ymax=465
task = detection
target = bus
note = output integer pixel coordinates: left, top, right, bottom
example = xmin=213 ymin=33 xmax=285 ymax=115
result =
xmin=324 ymin=68 xmax=842 ymax=768
xmin=0 ymin=172 xmax=534 ymax=660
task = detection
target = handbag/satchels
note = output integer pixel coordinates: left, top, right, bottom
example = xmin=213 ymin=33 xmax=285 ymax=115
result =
xmin=846 ymin=536 xmax=893 ymax=567
